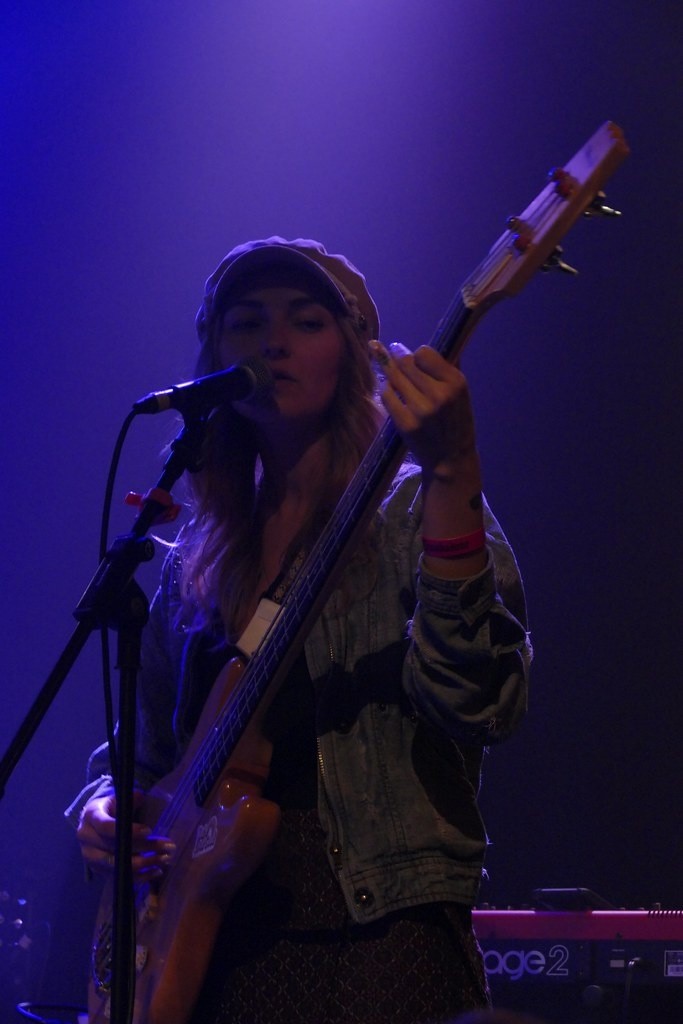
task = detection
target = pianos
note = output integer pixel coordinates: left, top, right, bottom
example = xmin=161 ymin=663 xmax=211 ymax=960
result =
xmin=471 ymin=904 xmax=682 ymax=1018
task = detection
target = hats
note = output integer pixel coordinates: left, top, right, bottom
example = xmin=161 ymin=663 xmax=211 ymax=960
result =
xmin=194 ymin=236 xmax=381 ymax=367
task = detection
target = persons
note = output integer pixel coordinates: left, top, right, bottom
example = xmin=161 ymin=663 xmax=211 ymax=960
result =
xmin=64 ymin=233 xmax=535 ymax=1022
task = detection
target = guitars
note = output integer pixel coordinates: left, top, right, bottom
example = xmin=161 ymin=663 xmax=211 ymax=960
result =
xmin=86 ymin=118 xmax=630 ymax=1022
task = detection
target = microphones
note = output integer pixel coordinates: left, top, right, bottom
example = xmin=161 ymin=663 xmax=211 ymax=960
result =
xmin=131 ymin=355 xmax=277 ymax=416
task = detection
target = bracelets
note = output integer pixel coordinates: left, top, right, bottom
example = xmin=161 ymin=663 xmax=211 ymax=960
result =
xmin=419 ymin=530 xmax=488 ymax=559
